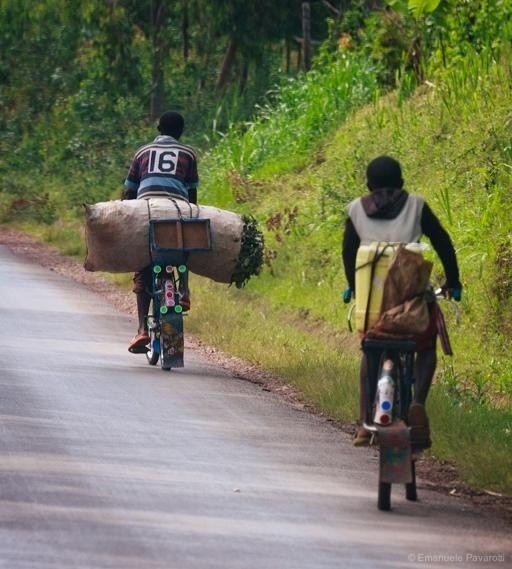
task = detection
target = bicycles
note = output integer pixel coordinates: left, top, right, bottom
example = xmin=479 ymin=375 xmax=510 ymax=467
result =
xmin=337 ymin=281 xmax=454 ymax=510
xmin=133 ymin=256 xmax=192 ymax=369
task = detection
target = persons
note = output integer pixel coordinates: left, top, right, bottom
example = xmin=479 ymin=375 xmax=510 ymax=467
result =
xmin=121 ymin=110 xmax=200 ymax=355
xmin=341 ymin=155 xmax=463 ymax=448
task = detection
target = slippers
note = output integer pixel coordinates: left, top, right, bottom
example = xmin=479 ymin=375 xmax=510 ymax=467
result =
xmin=178 ymin=300 xmax=190 ymax=312
xmin=407 ymin=403 xmax=433 ymax=450
xmin=128 ymin=334 xmax=150 ymax=350
xmin=350 ymin=430 xmax=371 ymax=446
